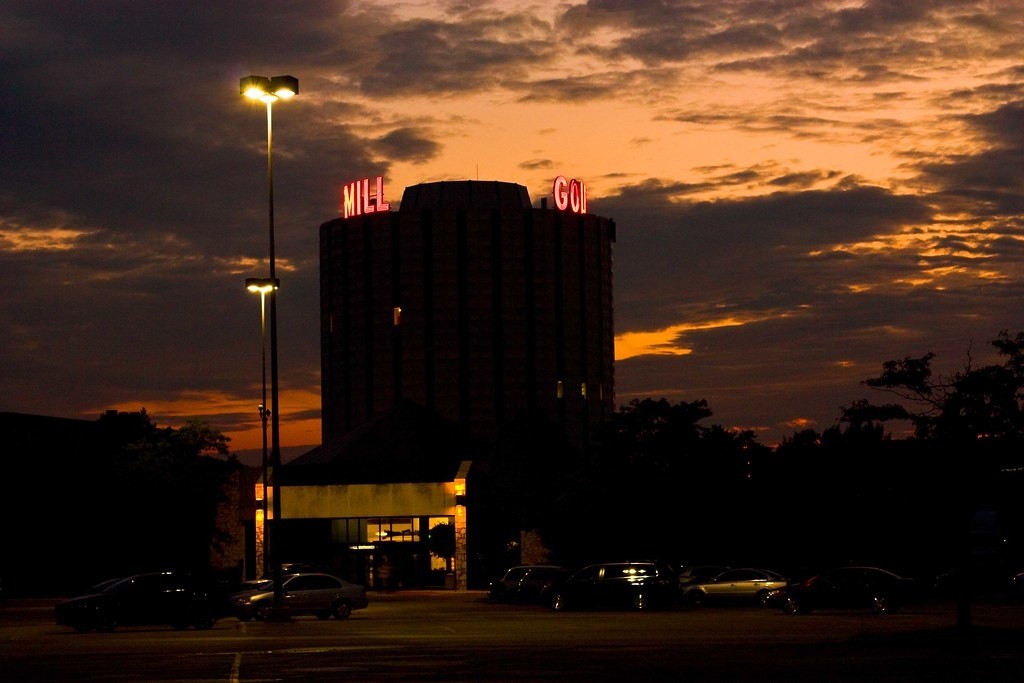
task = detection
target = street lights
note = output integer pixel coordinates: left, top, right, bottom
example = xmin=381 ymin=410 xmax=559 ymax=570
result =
xmin=238 ymin=74 xmax=300 ymax=580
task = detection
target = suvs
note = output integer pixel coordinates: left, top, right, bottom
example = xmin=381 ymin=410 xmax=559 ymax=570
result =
xmin=569 ymin=563 xmax=675 ymax=610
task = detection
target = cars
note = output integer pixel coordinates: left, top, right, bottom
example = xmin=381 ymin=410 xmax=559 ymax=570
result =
xmin=761 ymin=563 xmax=924 ymax=615
xmin=231 ymin=572 xmax=369 ymax=621
xmin=52 ymin=571 xmax=229 ymax=631
xmin=485 ymin=565 xmax=562 ymax=603
xmin=679 ymin=564 xmax=803 ymax=606
